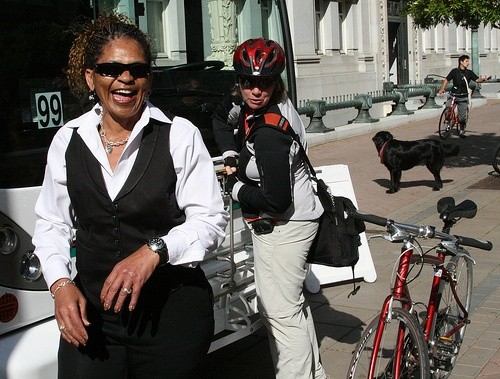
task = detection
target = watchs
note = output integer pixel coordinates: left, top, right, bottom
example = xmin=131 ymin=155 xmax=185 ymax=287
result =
xmin=147 ymin=236 xmax=169 ymax=265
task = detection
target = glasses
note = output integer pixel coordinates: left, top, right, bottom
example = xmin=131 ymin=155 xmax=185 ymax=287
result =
xmin=239 ymin=77 xmax=276 ymax=89
xmin=92 ymin=62 xmax=153 ymax=77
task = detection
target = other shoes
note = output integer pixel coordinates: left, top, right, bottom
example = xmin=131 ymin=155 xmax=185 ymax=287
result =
xmin=459 ymin=130 xmax=464 ymax=137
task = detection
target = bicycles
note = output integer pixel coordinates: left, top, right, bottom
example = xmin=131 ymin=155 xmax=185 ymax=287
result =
xmin=346 ymin=196 xmax=493 ymax=379
xmin=439 ymin=90 xmax=469 ymax=140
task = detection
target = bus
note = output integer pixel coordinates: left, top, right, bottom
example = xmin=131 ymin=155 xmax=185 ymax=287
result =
xmin=0 ymin=0 xmax=308 ymax=379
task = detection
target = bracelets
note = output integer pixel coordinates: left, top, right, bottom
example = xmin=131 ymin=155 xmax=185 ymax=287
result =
xmin=51 ymin=280 xmax=76 ymax=299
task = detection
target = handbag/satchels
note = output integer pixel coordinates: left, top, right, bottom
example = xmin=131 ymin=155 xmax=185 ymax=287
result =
xmin=306 ymin=179 xmax=366 ymax=267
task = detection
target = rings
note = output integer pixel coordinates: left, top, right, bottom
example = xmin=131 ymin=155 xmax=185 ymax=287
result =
xmin=121 ymin=287 xmax=132 ymax=295
xmin=58 ymin=326 xmax=66 ymax=331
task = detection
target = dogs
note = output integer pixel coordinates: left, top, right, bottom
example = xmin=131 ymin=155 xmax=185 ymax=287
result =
xmin=372 ymin=131 xmax=459 ymax=194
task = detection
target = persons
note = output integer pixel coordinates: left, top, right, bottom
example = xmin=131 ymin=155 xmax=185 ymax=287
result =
xmin=31 ymin=9 xmax=231 ymax=379
xmin=438 ymin=55 xmax=492 ymax=138
xmin=222 ymin=38 xmax=328 ymax=379
xmin=161 ymin=76 xmax=222 ymax=157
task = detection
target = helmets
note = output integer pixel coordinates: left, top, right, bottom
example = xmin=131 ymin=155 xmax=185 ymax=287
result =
xmin=233 ymin=38 xmax=285 ymax=77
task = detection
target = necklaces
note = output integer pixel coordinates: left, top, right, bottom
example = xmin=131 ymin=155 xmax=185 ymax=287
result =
xmin=99 ymin=121 xmax=130 ymax=154
